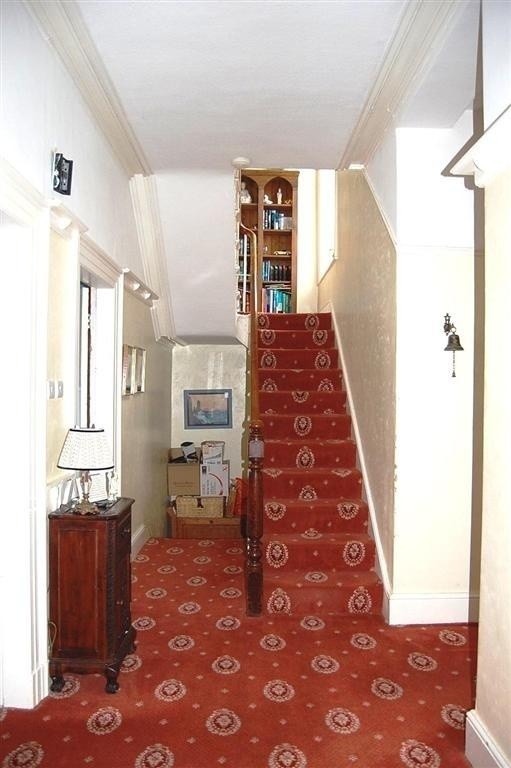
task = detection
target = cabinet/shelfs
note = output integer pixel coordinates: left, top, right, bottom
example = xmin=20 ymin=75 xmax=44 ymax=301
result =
xmin=46 ymin=497 xmax=138 ymax=695
xmin=238 ymin=169 xmax=301 ymax=316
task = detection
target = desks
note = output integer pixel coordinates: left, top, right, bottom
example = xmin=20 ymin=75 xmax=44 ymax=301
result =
xmin=164 ymin=501 xmax=241 ymax=539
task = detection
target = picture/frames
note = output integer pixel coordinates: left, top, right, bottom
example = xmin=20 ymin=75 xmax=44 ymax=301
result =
xmin=183 ymin=389 xmax=233 ymax=429
xmin=122 ymin=343 xmax=147 ymax=397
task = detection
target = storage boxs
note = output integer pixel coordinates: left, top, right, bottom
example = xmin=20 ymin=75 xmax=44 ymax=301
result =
xmin=168 ymin=440 xmax=231 ymax=506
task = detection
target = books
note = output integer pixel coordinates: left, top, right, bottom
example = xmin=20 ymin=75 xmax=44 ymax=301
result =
xmin=262 ymin=288 xmax=290 ymax=313
xmin=262 ymin=259 xmax=291 ymax=280
xmin=263 ymin=209 xmax=284 ymax=230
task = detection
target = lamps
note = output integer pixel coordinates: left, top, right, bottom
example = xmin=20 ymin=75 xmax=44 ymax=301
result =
xmin=56 ymin=423 xmax=114 ymax=515
